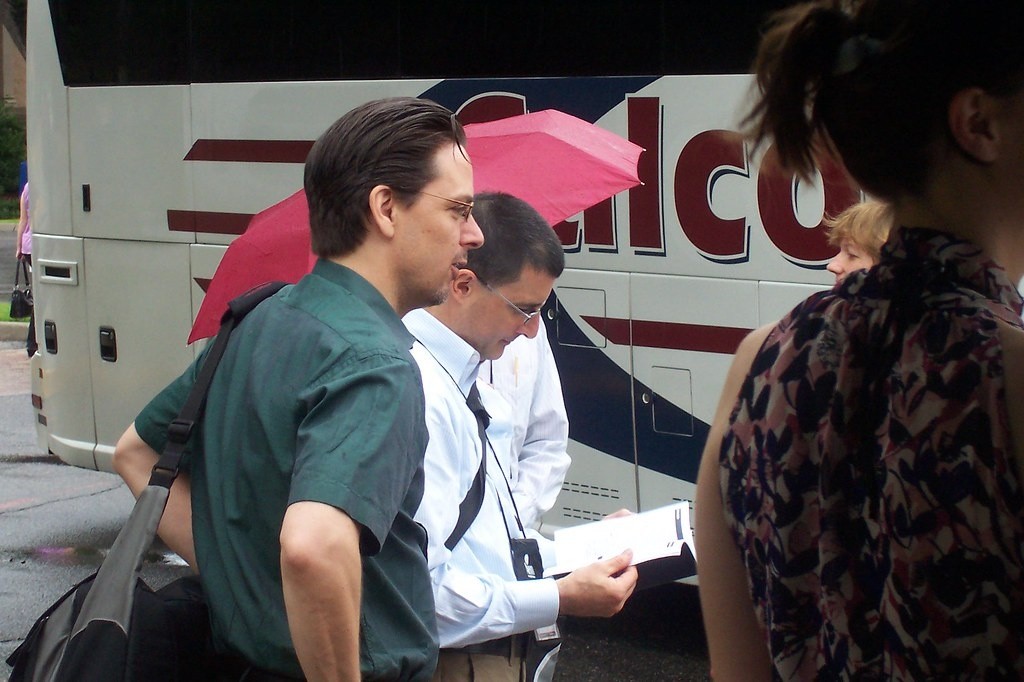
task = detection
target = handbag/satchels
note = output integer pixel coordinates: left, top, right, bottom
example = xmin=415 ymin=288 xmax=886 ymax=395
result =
xmin=10 ymin=253 xmax=34 ymax=318
xmin=6 ymin=282 xmax=289 ymax=682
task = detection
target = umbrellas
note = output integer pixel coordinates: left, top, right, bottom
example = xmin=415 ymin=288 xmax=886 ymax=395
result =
xmin=186 ymin=108 xmax=647 ymax=346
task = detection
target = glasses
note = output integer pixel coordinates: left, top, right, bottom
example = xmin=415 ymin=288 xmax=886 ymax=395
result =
xmin=463 ymin=267 xmax=547 ymax=324
xmin=386 ymin=184 xmax=475 ymax=222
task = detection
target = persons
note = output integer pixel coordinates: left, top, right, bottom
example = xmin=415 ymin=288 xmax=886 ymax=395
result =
xmin=540 ymin=632 xmax=554 ymax=639
xmin=477 ymin=313 xmax=572 ymax=532
xmin=826 ymin=201 xmax=894 ymax=291
xmin=16 ymin=182 xmax=38 ymax=358
xmin=402 ymin=190 xmax=638 ymax=682
xmin=692 ymin=0 xmax=1024 ymax=682
xmin=112 ymin=97 xmax=484 ymax=682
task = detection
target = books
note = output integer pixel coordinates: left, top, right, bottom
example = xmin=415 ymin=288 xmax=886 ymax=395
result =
xmin=542 ymin=499 xmax=699 ymax=587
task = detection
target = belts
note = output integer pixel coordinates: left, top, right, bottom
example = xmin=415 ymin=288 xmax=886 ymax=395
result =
xmin=440 ymin=631 xmax=536 ymax=661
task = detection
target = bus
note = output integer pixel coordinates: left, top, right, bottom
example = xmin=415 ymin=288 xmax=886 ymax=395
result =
xmin=25 ymin=0 xmax=889 ymax=586
